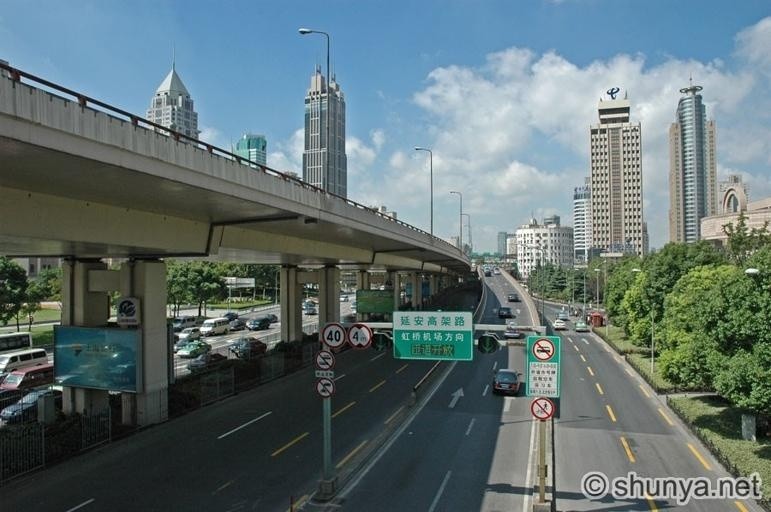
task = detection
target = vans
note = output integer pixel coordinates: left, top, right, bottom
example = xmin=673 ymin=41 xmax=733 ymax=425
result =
xmin=1 ymin=347 xmax=56 ymax=404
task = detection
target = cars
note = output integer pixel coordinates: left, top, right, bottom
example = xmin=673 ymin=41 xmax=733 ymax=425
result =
xmin=555 ymin=312 xmax=569 ymax=320
xmin=3 ymin=389 xmax=65 ymax=421
xmin=504 ymin=321 xmax=520 ymax=338
xmin=303 ymin=296 xmax=319 ymax=319
xmin=491 ymin=366 xmax=523 ymax=396
xmin=480 ymin=263 xmax=502 ymax=277
xmin=571 ymin=318 xmax=588 ymax=332
xmin=498 ymin=305 xmax=512 ymax=319
xmin=172 ymin=309 xmax=283 ymax=375
xmin=505 ymin=291 xmax=522 ymax=302
xmin=551 ymin=319 xmax=566 ymax=329
xmin=567 ymin=304 xmax=599 ymax=324
xmin=350 ymin=300 xmax=356 ymax=311
xmin=340 ymin=293 xmax=349 ymax=303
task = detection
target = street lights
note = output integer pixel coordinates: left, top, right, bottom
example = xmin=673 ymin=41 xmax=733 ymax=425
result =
xmin=297 ymin=27 xmax=330 ymax=194
xmin=412 ymin=145 xmax=434 ymax=242
xmin=450 ymin=191 xmax=463 ymax=250
xmin=457 ymin=212 xmax=471 ymax=250
xmin=625 ymin=266 xmax=657 ymax=374
xmin=532 ymin=245 xmax=607 ymax=312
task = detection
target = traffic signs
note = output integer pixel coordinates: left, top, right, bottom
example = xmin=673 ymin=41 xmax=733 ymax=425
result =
xmin=321 ymin=321 xmax=346 ymax=349
xmin=392 ymin=311 xmax=474 ymax=362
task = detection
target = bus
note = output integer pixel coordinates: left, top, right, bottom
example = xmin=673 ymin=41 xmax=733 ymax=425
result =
xmin=1 ymin=332 xmax=33 ymax=355
xmin=404 ymin=279 xmax=441 ymax=298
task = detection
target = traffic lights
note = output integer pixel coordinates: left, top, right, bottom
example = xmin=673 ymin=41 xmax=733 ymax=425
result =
xmin=369 ymin=331 xmax=393 ymax=353
xmin=479 ymin=332 xmax=497 ymax=355
